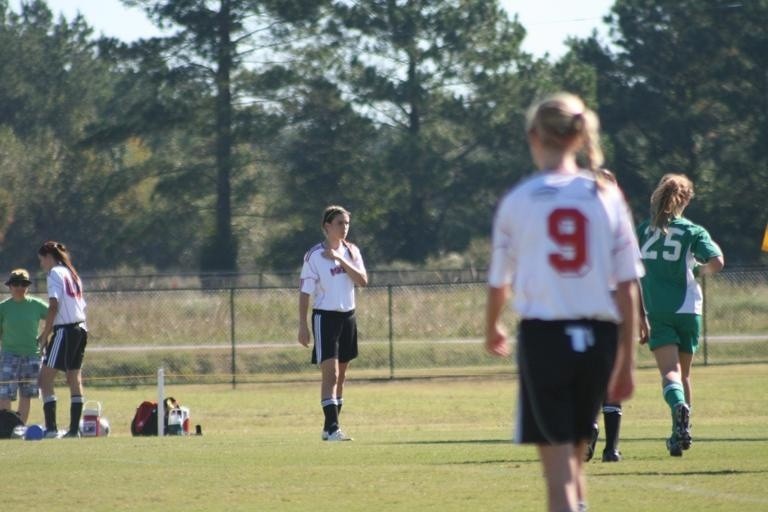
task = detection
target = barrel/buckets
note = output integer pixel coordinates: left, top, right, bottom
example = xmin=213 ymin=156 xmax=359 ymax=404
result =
xmin=81 ymin=400 xmax=102 ymax=436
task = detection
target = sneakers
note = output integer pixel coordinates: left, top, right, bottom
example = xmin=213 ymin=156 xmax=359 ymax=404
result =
xmin=602 ymin=449 xmax=621 ymax=462
xmin=328 ymin=428 xmax=354 ymax=441
xmin=667 ymin=402 xmax=689 ymax=458
xmin=42 ymin=430 xmax=59 ymax=439
xmin=584 ymin=422 xmax=600 ymax=464
xmin=321 ymin=430 xmax=329 ymax=441
xmin=682 ymin=434 xmax=692 ymax=451
xmin=61 ymin=431 xmax=80 ymax=439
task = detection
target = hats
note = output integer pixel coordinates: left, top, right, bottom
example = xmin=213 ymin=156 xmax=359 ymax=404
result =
xmin=4 ymin=269 xmax=33 ymax=286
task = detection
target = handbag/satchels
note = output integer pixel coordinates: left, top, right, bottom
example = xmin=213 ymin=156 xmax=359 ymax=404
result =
xmin=130 ymin=396 xmax=184 ymax=437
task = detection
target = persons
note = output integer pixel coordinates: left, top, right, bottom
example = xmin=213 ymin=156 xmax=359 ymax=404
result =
xmin=582 ymin=167 xmax=651 ymax=462
xmin=36 ymin=240 xmax=89 ymax=438
xmin=299 ymin=205 xmax=369 ymax=443
xmin=484 ymin=91 xmax=647 ymax=511
xmin=0 ymin=269 xmax=50 ymax=424
xmin=638 ymin=172 xmax=724 ymax=457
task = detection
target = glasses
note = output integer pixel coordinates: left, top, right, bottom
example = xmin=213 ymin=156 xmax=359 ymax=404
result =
xmin=12 ymin=282 xmax=29 ymax=288
